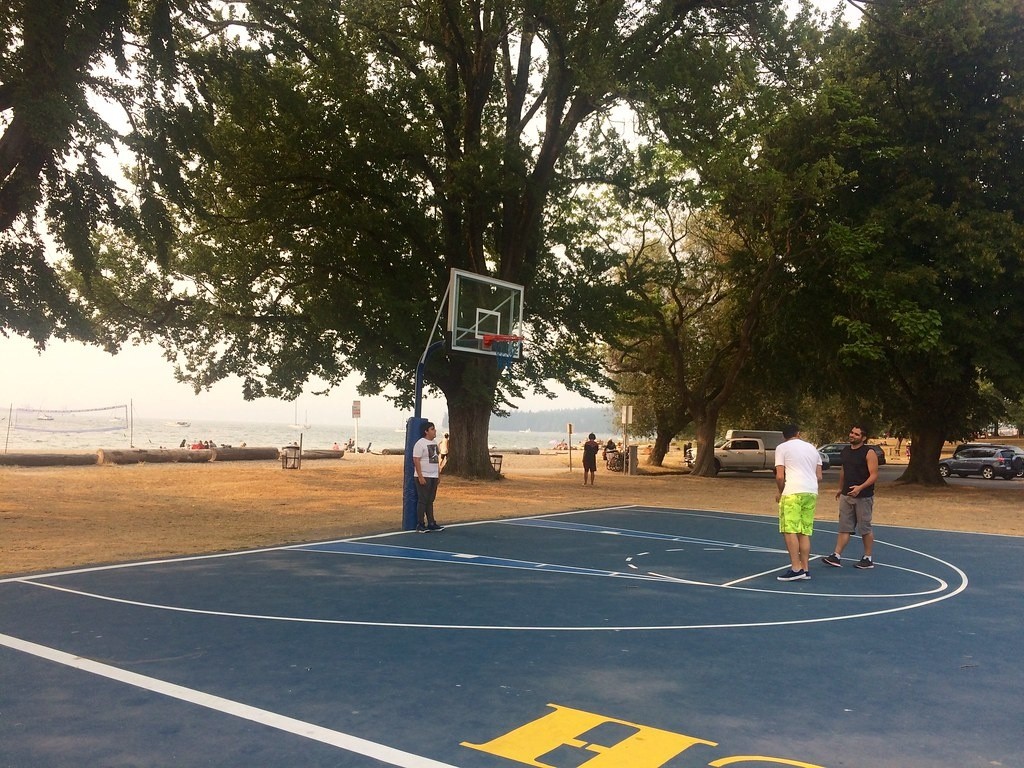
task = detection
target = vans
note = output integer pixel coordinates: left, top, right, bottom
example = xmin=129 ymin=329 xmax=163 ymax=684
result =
xmin=952 ymin=443 xmax=1024 ymax=477
xmin=726 ymin=428 xmax=831 ymax=470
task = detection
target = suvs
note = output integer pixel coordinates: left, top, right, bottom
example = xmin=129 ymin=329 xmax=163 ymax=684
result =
xmin=938 ymin=446 xmax=1023 ymax=480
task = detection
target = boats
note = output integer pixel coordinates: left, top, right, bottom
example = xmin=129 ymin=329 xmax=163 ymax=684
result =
xmin=37 ymin=415 xmax=54 ymax=420
xmin=517 ymin=428 xmax=533 ymax=435
xmin=395 ymin=427 xmax=406 ymax=433
xmin=167 ymin=421 xmax=192 ymax=428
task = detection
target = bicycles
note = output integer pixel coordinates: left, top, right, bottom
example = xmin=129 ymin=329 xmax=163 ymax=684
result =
xmin=604 ymin=447 xmax=639 ymax=472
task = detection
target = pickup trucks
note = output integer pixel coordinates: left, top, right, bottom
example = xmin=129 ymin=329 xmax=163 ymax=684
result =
xmin=684 ymin=437 xmax=777 ymax=474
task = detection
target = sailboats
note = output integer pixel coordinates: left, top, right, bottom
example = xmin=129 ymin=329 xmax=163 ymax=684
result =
xmin=289 ymin=396 xmax=312 ymax=430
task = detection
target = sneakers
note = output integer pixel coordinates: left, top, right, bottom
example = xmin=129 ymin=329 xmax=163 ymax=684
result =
xmin=777 ymin=569 xmax=806 ymax=581
xmin=802 ymin=572 xmax=811 ymax=580
xmin=417 ymin=524 xmax=430 ymax=533
xmin=854 ymin=556 xmax=874 ymax=569
xmin=427 ymin=523 xmax=445 ymax=531
xmin=822 ymin=554 xmax=841 ymax=567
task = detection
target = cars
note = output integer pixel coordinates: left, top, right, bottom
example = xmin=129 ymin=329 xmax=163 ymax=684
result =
xmin=817 ymin=443 xmax=887 ymax=466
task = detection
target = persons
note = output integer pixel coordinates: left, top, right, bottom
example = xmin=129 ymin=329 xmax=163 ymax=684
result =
xmin=179 ymin=439 xmax=217 ymax=449
xmin=822 ymin=425 xmax=878 ymax=569
xmin=582 ymin=433 xmax=599 ymax=486
xmin=413 ymin=422 xmax=444 ymax=533
xmin=343 ymin=438 xmax=355 ymax=451
xmin=438 ymin=433 xmax=449 ymax=467
xmin=774 ymin=424 xmax=822 ymax=581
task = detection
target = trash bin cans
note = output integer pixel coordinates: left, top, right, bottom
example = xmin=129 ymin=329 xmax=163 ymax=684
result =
xmin=489 ymin=454 xmax=503 ymax=473
xmin=281 ymin=446 xmax=300 ymax=469
